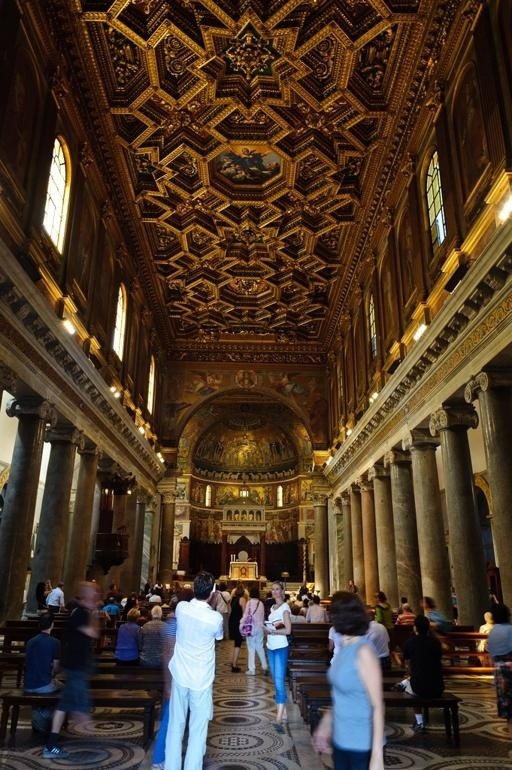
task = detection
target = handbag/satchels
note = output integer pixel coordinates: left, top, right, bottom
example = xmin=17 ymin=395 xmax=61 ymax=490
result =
xmin=240 ymin=615 xmax=254 ymax=637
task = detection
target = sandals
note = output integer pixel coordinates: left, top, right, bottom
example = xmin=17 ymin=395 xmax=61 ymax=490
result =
xmin=151 ymin=762 xmax=165 ymax=770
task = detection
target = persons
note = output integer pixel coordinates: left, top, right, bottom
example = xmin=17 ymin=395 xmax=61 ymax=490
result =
xmin=277 ymin=373 xmax=301 ymax=394
xmin=23 ymin=573 xmax=292 ymax=770
xmin=222 ymin=148 xmax=278 ymax=183
xmin=240 ymin=369 xmax=254 ymax=388
xmin=196 ymin=374 xmax=224 ymax=395
xmin=293 ymin=581 xmax=512 ymax=770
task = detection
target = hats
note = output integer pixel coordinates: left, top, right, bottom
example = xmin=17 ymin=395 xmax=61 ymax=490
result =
xmin=152 ymin=606 xmax=162 ymax=618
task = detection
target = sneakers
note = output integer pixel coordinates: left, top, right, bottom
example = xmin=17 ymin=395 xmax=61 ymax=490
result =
xmin=245 ymin=670 xmax=255 ymax=675
xmin=231 ymin=666 xmax=241 ymax=672
xmin=409 ymin=721 xmax=425 ymax=730
xmin=263 ymin=670 xmax=269 ymax=675
xmin=391 ymin=682 xmax=405 ymax=692
xmin=43 ymin=745 xmax=69 ymax=758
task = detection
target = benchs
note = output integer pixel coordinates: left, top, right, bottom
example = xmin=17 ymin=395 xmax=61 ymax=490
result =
xmin=279 ymin=597 xmax=512 ymax=752
xmin=1 ymin=601 xmax=174 ymax=752
